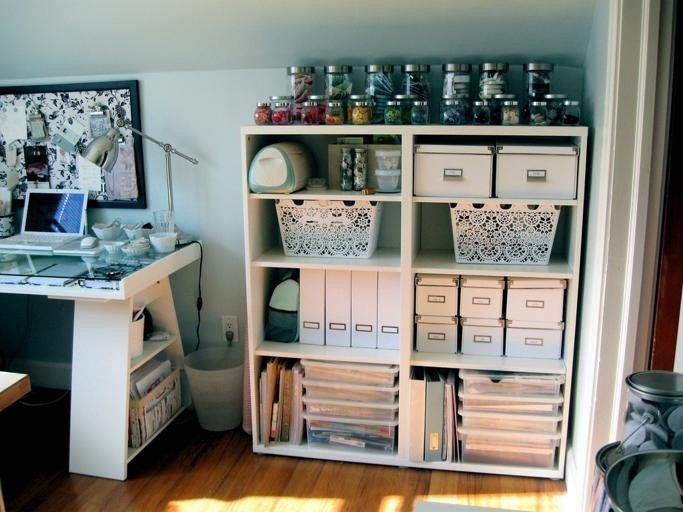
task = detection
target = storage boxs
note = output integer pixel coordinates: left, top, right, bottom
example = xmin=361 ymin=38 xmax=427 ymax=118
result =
xmin=416 ymin=317 xmax=458 ymax=353
xmin=128 ymin=366 xmax=181 ymax=449
xmin=505 ymin=324 xmax=564 ymax=359
xmin=415 ymin=274 xmax=460 ymax=316
xmin=507 ymin=280 xmax=568 ymax=324
xmin=495 ymin=146 xmax=578 ymax=199
xmin=459 ymin=320 xmax=505 ymax=355
xmin=302 ymin=360 xmax=401 ymax=450
xmin=413 ymin=144 xmax=497 ymax=200
xmin=461 ymin=276 xmax=506 ymax=317
xmin=328 ymin=141 xmax=403 ymax=191
xmin=455 ymin=373 xmax=563 ymax=468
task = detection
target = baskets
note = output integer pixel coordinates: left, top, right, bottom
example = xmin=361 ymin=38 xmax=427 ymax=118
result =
xmin=275 ymin=199 xmax=384 ymax=259
xmin=449 ymin=200 xmax=562 ymax=265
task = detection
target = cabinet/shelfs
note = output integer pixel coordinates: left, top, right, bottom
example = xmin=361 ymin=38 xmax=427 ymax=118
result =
xmin=240 ymin=126 xmax=588 ymax=484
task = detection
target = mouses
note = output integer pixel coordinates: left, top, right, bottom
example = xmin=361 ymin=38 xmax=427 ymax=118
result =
xmin=81 ymin=236 xmax=98 ymax=248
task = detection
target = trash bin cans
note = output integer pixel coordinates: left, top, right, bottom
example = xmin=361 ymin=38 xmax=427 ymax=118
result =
xmin=184 ymin=346 xmax=243 ymax=431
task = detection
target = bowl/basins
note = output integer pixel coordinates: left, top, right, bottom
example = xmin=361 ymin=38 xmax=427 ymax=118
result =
xmin=91 ymin=223 xmax=177 ymax=256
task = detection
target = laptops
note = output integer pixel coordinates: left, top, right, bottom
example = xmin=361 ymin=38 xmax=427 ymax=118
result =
xmin=0 ymin=189 xmax=89 ymax=251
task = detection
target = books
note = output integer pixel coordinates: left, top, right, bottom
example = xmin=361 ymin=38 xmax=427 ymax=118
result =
xmin=130 ymin=359 xmax=172 ymax=400
xmin=259 ymin=357 xmax=305 ymax=447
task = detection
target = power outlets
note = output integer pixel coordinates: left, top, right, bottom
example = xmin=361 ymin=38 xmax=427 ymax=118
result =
xmin=221 ymin=315 xmax=240 ymax=345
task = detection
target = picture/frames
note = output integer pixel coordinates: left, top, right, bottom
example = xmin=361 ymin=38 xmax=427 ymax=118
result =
xmin=0 ymin=79 xmax=147 ymax=209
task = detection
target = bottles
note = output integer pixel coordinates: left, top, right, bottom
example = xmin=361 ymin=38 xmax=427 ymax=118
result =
xmin=253 ymin=63 xmax=581 ymax=126
xmin=341 ymin=147 xmax=400 ymax=191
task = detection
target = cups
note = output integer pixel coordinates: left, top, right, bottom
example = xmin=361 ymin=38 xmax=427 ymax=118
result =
xmin=0 ymin=214 xmax=14 ymax=238
xmin=152 ymin=210 xmax=175 ymax=233
xmin=131 ymin=311 xmax=145 ymax=358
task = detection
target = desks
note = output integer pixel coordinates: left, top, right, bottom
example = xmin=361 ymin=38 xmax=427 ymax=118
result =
xmin=0 ymin=239 xmax=202 ymax=483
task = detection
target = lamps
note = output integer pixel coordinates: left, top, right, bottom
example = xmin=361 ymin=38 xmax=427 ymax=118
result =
xmin=82 ymin=119 xmax=197 ymax=254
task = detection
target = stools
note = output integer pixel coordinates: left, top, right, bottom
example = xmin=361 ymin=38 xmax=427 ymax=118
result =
xmin=2 ymin=372 xmax=31 ymax=508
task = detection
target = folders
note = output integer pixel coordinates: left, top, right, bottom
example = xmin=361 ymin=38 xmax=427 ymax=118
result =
xmin=424 ymin=371 xmax=445 ymax=461
xmin=411 ymin=369 xmax=427 ymax=460
